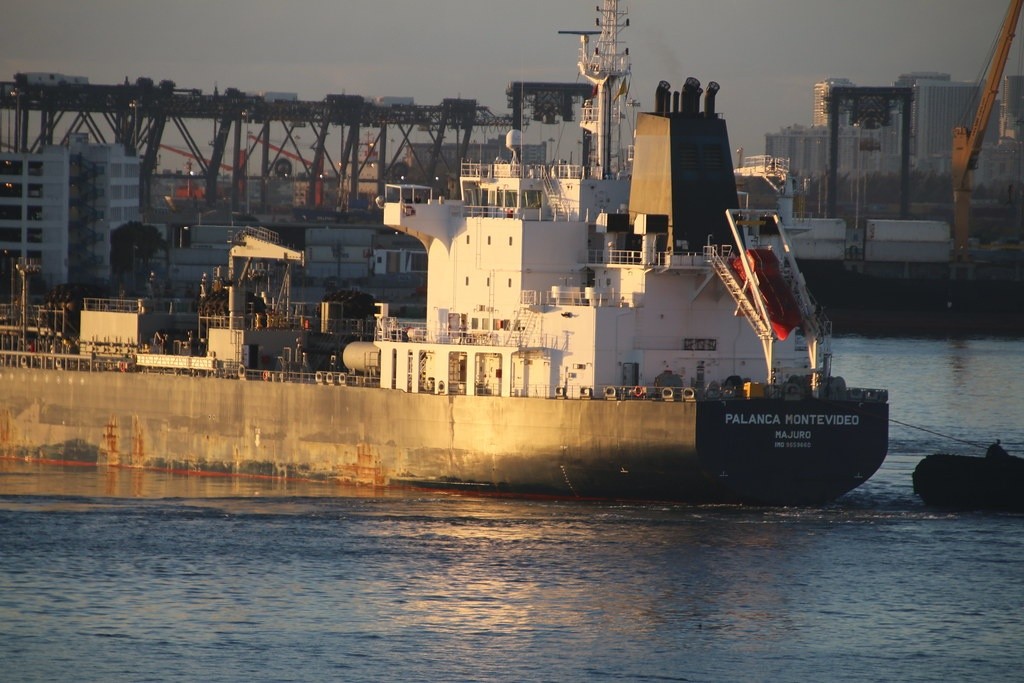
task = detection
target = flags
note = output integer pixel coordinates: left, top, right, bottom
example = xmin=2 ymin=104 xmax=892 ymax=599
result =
xmin=614 ymin=77 xmax=627 ymax=103
xmin=592 ymin=85 xmax=598 ymax=96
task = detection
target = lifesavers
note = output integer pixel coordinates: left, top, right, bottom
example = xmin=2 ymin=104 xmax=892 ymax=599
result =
xmin=237 ymin=365 xmax=246 ymax=378
xmin=338 ymin=373 xmax=347 ymax=384
xmin=21 ymin=355 xmax=27 ymax=366
xmin=121 ymin=362 xmax=125 ymax=372
xmin=662 ymin=389 xmax=674 ymax=399
xmin=315 ymin=372 xmax=324 ymax=382
xmin=579 ymin=387 xmax=592 ymax=396
xmin=56 ymin=358 xmax=63 ymax=368
xmin=554 ymin=386 xmax=566 ymax=397
xmin=604 ymin=386 xmax=616 ymax=397
xmin=634 ymin=386 xmax=642 ymax=396
xmin=683 ymin=389 xmax=694 ymax=399
xmin=326 ymin=373 xmax=335 ymax=383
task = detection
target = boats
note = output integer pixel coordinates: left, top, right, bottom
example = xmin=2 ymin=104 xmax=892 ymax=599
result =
xmin=1 ymin=0 xmax=891 ymax=507
xmin=912 ymin=449 xmax=1024 ymax=510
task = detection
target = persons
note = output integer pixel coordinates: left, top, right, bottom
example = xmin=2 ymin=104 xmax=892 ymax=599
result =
xmin=415 ymin=193 xmax=421 ymax=202
xmin=986 ymin=439 xmax=1007 ymax=457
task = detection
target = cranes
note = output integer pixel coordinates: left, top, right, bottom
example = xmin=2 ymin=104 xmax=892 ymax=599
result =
xmin=952 ymin=0 xmax=1023 ymax=254
xmin=142 ymin=138 xmax=232 ymax=195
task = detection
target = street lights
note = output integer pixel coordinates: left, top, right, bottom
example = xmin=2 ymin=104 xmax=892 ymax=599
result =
xmin=8 ymin=86 xmax=29 ymax=155
xmin=240 ymin=108 xmax=256 ymax=216
xmin=127 ymin=98 xmax=143 ymax=143
